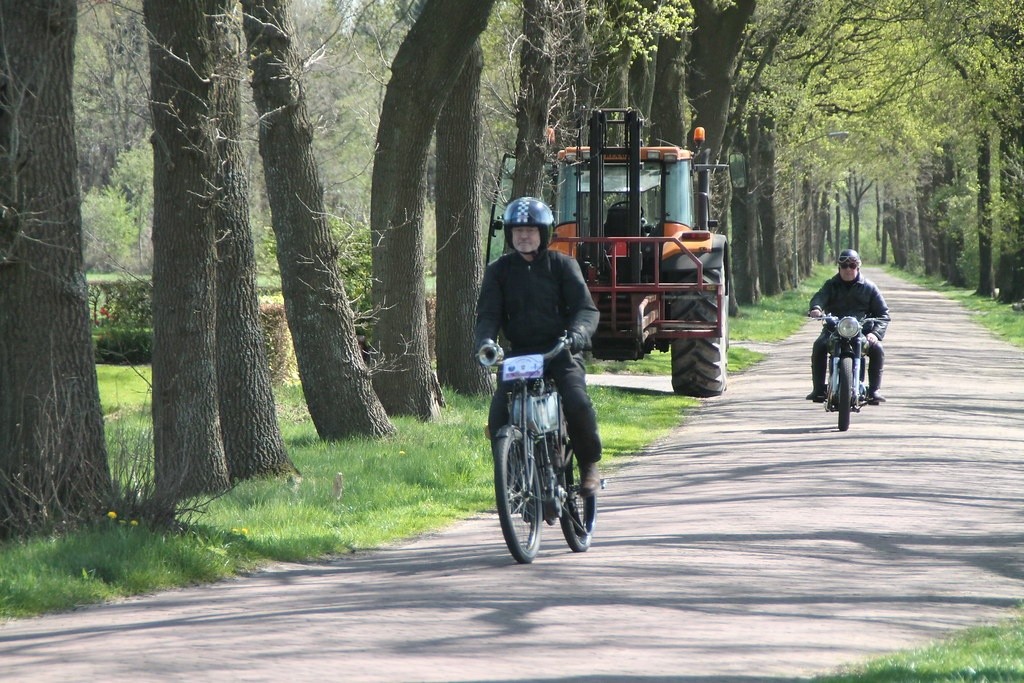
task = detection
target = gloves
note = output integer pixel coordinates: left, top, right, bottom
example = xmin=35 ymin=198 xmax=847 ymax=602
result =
xmin=561 ymin=331 xmax=586 ymax=355
xmin=482 ymin=338 xmax=505 ymax=362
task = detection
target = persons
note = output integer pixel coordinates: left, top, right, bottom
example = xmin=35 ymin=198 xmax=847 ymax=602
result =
xmin=473 ymin=197 xmax=602 ymax=523
xmin=805 ymin=250 xmax=891 ymax=404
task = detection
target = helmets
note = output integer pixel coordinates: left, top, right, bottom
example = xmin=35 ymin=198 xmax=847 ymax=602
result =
xmin=837 ymin=249 xmax=861 ymax=264
xmin=502 ymin=196 xmax=554 ymax=255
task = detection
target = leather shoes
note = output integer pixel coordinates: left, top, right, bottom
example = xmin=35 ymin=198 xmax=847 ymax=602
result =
xmin=578 ymin=462 xmax=601 ymax=498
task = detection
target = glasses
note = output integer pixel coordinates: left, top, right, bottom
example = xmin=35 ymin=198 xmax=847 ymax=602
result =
xmin=839 ymin=263 xmax=858 ymax=269
xmin=839 ymin=255 xmax=858 ymax=263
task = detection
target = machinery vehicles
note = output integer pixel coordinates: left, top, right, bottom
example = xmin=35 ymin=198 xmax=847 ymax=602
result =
xmin=485 ymin=107 xmax=732 ymax=397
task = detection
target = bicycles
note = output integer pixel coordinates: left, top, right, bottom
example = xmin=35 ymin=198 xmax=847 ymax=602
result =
xmin=475 ymin=330 xmax=607 ymax=562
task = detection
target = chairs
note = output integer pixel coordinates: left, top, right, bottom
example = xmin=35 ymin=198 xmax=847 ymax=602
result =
xmin=604 ymin=200 xmax=645 ymax=236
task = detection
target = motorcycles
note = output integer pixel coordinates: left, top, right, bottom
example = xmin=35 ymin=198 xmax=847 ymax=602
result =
xmin=811 ymin=309 xmax=890 ymax=432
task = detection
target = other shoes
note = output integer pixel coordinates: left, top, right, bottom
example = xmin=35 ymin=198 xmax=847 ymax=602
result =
xmin=868 ymin=388 xmax=886 ymax=403
xmin=806 ymin=387 xmax=829 ymax=400
